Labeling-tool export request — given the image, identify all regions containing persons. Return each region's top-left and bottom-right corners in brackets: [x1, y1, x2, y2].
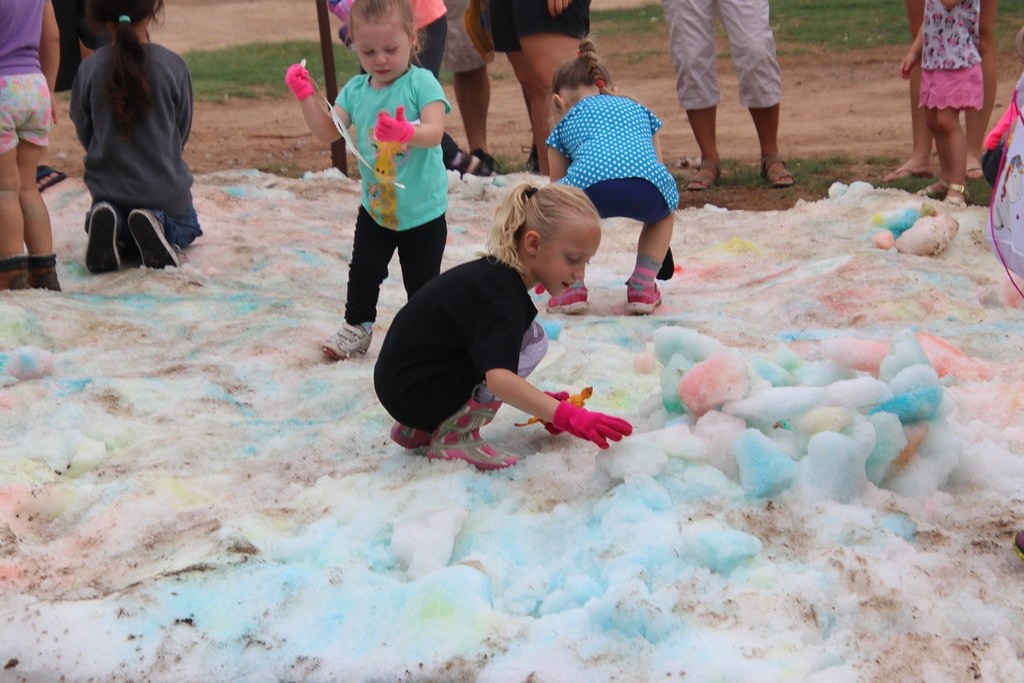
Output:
[410, 0, 593, 177]
[67, 0, 205, 273]
[0, 0, 62, 292]
[882, 0, 999, 183]
[285, 0, 452, 360]
[373, 184, 632, 472]
[545, 40, 680, 313]
[660, 0, 795, 190]
[903, 0, 984, 208]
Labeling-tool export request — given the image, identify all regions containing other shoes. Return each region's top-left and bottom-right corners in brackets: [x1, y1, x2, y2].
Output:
[127, 209, 179, 269]
[522, 144, 538, 172]
[86, 201, 121, 274]
[473, 149, 501, 176]
[0, 254, 28, 289]
[27, 253, 60, 291]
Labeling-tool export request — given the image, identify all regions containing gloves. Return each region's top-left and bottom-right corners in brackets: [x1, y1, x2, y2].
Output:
[285, 64, 316, 101]
[539, 390, 570, 436]
[554, 399, 633, 449]
[376, 105, 415, 144]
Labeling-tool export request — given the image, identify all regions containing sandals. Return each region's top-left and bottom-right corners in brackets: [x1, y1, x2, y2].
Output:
[917, 179, 949, 198]
[945, 185, 967, 206]
[760, 156, 794, 187]
[689, 160, 721, 190]
[36, 165, 65, 191]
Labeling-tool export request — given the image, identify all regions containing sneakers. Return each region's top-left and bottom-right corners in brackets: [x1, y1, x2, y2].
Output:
[627, 282, 661, 314]
[546, 287, 588, 315]
[319, 321, 372, 359]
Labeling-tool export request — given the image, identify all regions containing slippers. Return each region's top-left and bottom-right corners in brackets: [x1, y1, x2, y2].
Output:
[885, 168, 922, 182]
[966, 162, 984, 179]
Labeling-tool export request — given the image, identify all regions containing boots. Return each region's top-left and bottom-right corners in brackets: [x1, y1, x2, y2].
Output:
[428, 398, 518, 470]
[390, 420, 436, 449]
